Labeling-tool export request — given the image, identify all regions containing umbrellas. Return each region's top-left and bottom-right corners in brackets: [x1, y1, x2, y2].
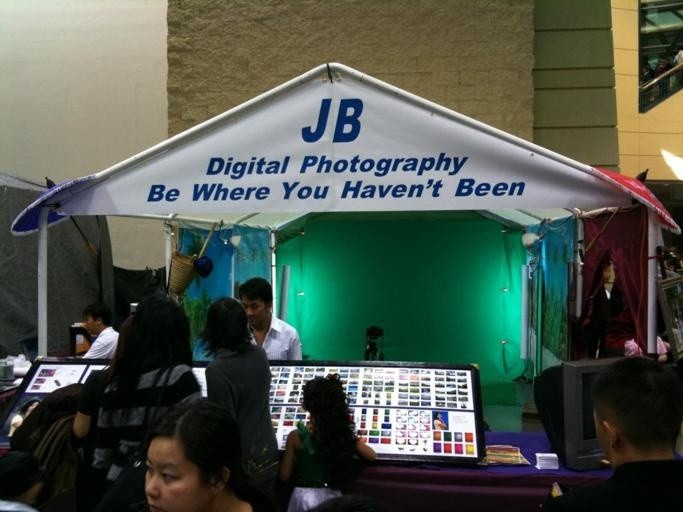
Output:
[580, 155, 680, 262]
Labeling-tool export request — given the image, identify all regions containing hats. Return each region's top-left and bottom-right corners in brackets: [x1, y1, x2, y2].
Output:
[1, 450, 47, 498]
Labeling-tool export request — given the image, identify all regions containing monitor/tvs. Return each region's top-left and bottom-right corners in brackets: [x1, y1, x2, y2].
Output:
[532, 357, 632, 470]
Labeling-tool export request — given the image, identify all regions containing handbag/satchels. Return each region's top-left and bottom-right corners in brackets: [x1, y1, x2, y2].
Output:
[168, 255, 196, 295]
[97, 362, 175, 511]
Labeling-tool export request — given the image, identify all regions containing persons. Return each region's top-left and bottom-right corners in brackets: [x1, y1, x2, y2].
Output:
[431, 411, 448, 431]
[237, 276, 304, 361]
[541, 356, 682, 512]
[73, 293, 203, 512]
[282, 374, 376, 489]
[196, 295, 280, 512]
[134, 394, 281, 512]
[0, 451, 48, 512]
[4, 395, 39, 438]
[77, 301, 120, 361]
